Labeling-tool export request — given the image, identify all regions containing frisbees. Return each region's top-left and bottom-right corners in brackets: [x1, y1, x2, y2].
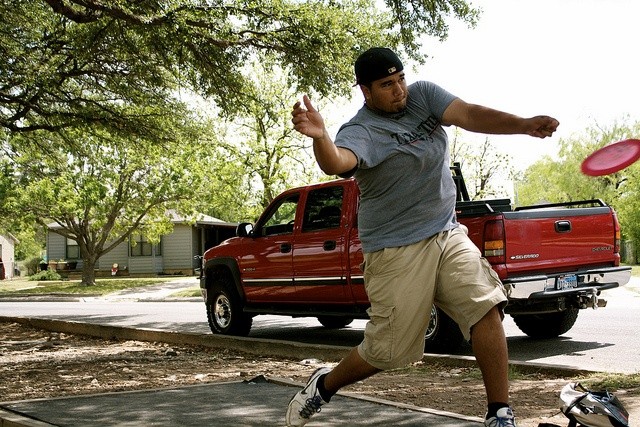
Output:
[580, 138, 640, 176]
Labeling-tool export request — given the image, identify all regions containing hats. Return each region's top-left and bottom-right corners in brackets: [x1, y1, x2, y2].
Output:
[352, 46, 404, 88]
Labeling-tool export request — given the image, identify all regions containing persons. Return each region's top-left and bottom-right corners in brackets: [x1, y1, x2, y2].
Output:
[286, 47, 559, 427]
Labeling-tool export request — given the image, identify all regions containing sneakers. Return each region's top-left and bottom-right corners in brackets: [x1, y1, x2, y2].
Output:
[483, 407, 518, 427]
[285, 364, 332, 426]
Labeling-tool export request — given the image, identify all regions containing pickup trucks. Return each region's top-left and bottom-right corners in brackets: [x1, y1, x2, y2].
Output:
[194, 163, 632, 353]
